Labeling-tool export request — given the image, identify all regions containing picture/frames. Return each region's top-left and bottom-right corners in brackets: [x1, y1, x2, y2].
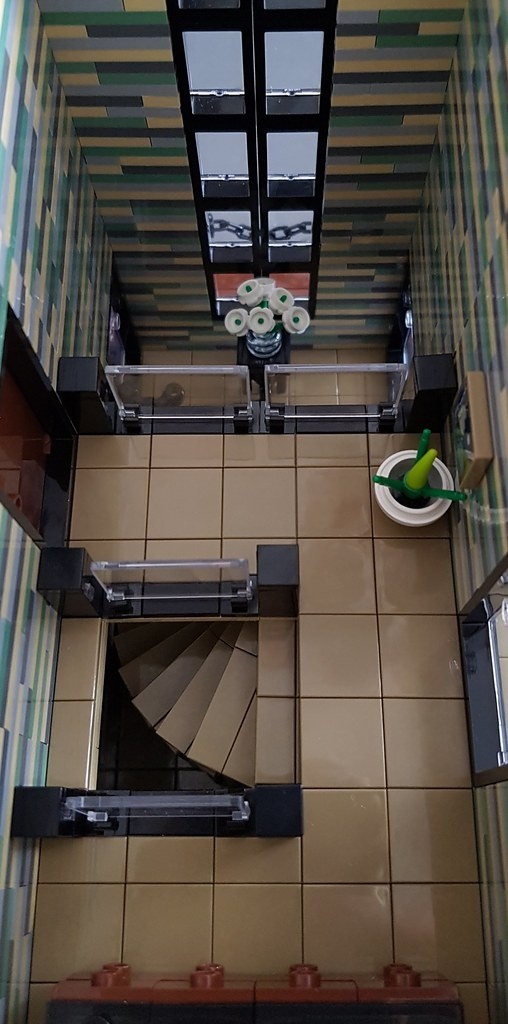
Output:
[451, 369, 494, 490]
